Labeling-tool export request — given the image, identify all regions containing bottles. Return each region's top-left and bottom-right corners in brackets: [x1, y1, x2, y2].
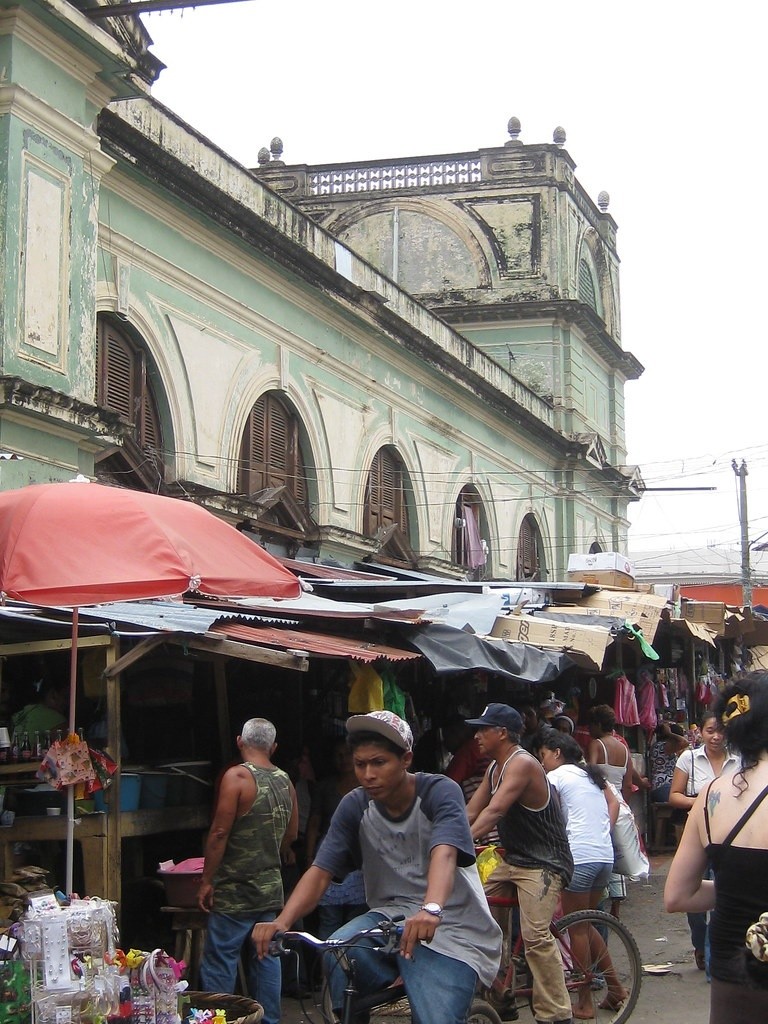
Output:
[33, 731, 41, 762]
[57, 730, 62, 742]
[11, 733, 21, 763]
[42, 730, 50, 761]
[21, 732, 31, 763]
[78, 727, 85, 742]
[0, 747, 11, 764]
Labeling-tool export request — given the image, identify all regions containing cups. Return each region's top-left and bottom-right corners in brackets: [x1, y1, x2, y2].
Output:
[0, 727, 10, 747]
[47, 808, 61, 816]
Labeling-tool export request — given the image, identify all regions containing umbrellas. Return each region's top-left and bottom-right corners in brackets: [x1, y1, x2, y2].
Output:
[3, 473, 301, 894]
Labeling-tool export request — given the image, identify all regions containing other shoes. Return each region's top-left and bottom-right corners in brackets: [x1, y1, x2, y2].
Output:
[495, 1006, 519, 1022]
[695, 947, 705, 970]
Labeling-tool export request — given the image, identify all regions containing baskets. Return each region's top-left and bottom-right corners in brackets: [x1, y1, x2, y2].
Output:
[101, 991, 264, 1024]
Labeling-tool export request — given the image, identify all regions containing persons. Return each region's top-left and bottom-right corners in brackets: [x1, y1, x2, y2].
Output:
[252, 702, 649, 1023]
[196, 718, 300, 1023]
[647, 672, 768, 1023]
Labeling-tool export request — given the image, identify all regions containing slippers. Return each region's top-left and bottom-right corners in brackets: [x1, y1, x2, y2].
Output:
[596, 1000, 626, 1009]
[571, 1004, 595, 1019]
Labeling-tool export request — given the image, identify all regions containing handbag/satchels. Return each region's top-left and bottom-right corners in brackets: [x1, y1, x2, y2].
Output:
[475, 844, 506, 886]
[603, 778, 650, 882]
[694, 677, 713, 706]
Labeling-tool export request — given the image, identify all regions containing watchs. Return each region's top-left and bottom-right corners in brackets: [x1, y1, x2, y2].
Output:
[421, 901, 446, 919]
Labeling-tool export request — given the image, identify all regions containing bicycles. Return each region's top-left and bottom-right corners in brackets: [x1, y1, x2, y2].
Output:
[474, 837, 644, 1024]
[269, 911, 502, 1024]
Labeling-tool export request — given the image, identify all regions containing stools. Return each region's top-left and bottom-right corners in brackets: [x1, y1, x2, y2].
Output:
[652, 802, 677, 855]
[160, 906, 250, 998]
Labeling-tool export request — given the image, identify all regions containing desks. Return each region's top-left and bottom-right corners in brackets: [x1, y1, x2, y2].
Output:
[0, 803, 212, 928]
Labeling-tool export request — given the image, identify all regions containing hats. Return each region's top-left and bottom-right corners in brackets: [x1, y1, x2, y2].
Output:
[346, 709, 414, 751]
[465, 703, 524, 733]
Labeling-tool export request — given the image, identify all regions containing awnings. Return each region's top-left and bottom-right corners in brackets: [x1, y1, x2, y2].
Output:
[2, 585, 768, 673]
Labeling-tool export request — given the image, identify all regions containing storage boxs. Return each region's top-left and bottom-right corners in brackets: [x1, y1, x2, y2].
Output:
[568, 570, 634, 589]
[568, 551, 636, 579]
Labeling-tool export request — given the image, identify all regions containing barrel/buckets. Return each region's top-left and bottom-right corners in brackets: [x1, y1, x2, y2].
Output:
[94, 772, 141, 812]
